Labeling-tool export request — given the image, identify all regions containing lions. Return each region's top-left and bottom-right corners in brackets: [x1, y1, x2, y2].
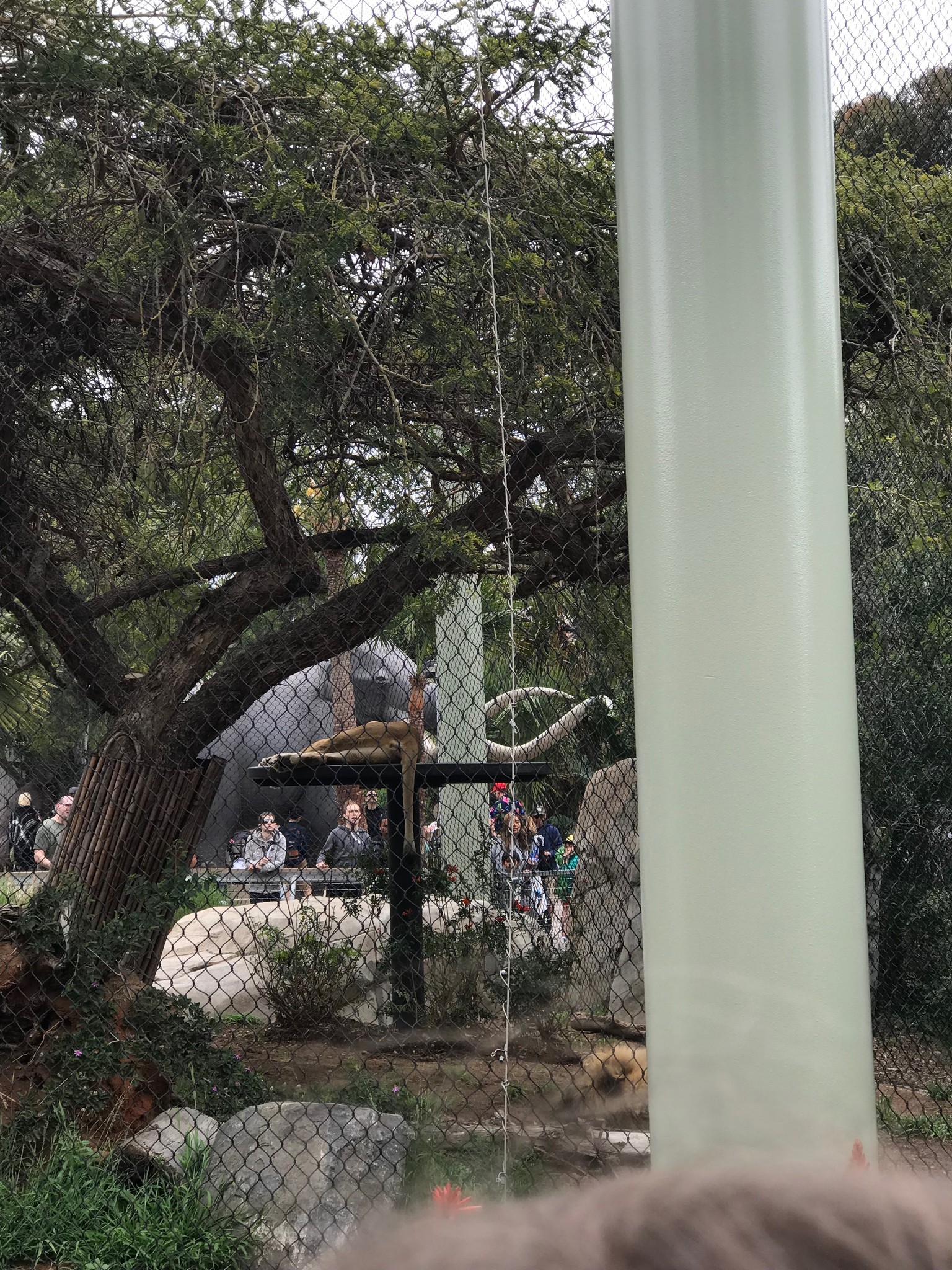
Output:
[258, 721, 437, 866]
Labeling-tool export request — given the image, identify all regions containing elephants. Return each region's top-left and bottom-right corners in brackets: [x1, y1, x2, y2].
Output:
[183, 635, 614, 869]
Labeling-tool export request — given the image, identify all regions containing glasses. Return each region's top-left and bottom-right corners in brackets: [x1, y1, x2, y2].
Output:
[534, 814, 545, 818]
[264, 819, 275, 823]
[367, 794, 378, 797]
[59, 803, 71, 808]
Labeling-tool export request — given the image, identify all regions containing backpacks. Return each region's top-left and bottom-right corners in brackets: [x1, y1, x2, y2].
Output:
[285, 830, 303, 860]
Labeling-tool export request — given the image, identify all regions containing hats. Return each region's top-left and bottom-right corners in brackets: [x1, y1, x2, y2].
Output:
[534, 805, 546, 815]
[563, 835, 573, 844]
[18, 792, 32, 806]
[490, 783, 506, 793]
[69, 786, 78, 797]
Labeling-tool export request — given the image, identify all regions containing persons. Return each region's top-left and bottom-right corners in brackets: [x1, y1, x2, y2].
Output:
[281, 806, 313, 900]
[189, 854, 202, 869]
[244, 811, 286, 904]
[316, 800, 372, 899]
[33, 787, 78, 871]
[8, 792, 43, 872]
[489, 783, 579, 935]
[364, 789, 442, 868]
